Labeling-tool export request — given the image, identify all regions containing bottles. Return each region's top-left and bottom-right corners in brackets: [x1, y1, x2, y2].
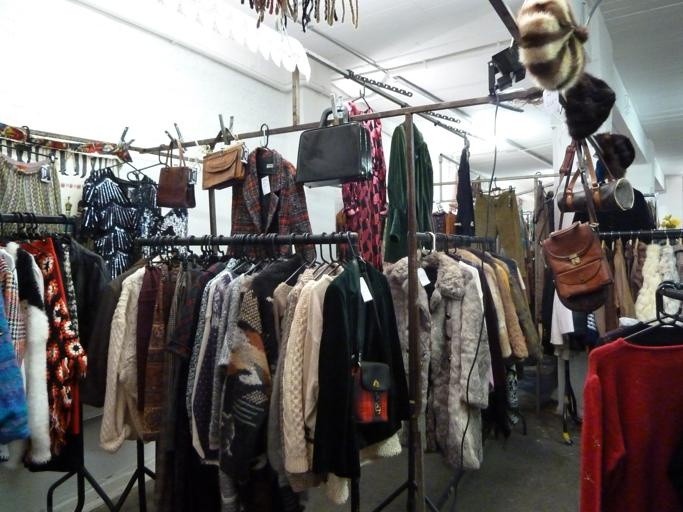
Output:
[594, 135, 635, 178]
[516, 0, 586, 93]
[569, 73, 614, 138]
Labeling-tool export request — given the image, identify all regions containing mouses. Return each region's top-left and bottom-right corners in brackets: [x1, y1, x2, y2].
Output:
[104, 142, 171, 185]
[617, 278, 683, 340]
[131, 234, 366, 288]
[596, 226, 683, 253]
[420, 234, 517, 273]
[252, 123, 274, 164]
[0, 211, 75, 259]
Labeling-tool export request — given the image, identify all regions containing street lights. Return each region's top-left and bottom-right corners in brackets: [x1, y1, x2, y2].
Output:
[294, 107, 373, 188]
[541, 223, 612, 314]
[350, 272, 392, 426]
[157, 139, 196, 206]
[558, 137, 635, 212]
[203, 129, 247, 189]
